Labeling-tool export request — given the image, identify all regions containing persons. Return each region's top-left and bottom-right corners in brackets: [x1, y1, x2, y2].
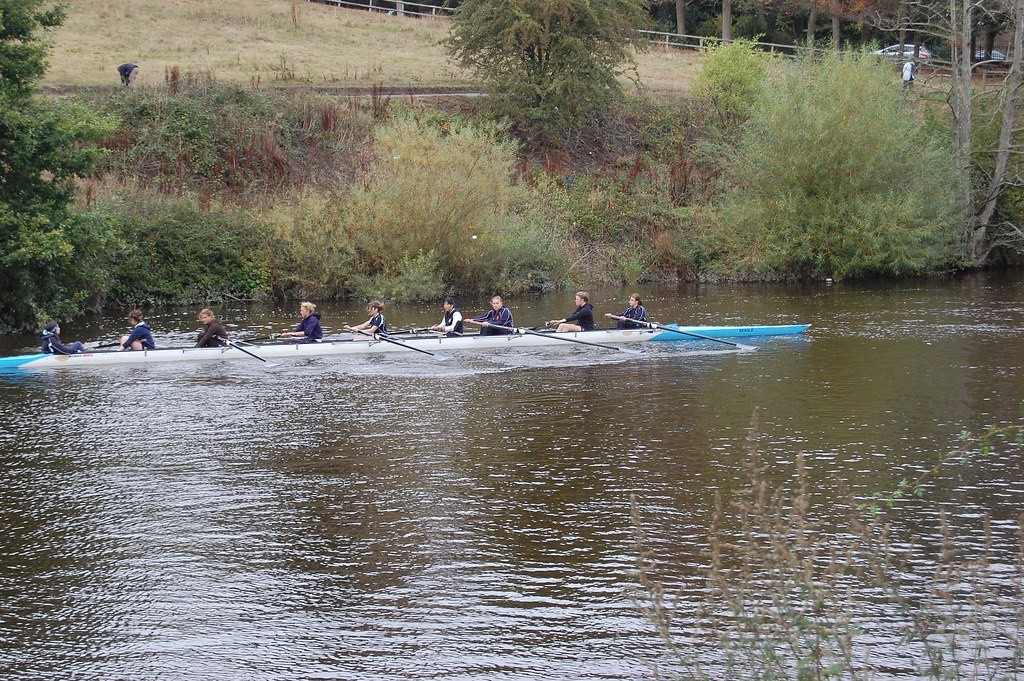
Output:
[350, 300, 388, 342]
[605, 292, 647, 329]
[464, 292, 514, 336]
[901, 56, 915, 92]
[281, 300, 323, 344]
[116, 307, 155, 351]
[41, 321, 87, 353]
[117, 63, 139, 89]
[430, 296, 464, 338]
[194, 307, 229, 348]
[549, 290, 594, 332]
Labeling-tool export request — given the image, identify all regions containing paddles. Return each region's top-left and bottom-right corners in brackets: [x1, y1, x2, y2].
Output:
[234, 334, 281, 343]
[606, 312, 761, 351]
[464, 318, 642, 353]
[388, 325, 439, 335]
[91, 341, 120, 350]
[214, 334, 276, 364]
[524, 320, 555, 329]
[345, 324, 449, 359]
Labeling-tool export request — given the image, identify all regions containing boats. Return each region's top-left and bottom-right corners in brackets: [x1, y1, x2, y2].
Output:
[1, 324, 813, 377]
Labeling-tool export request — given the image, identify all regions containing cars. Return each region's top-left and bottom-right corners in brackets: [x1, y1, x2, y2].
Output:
[870, 44, 932, 65]
[958, 50, 1008, 65]
